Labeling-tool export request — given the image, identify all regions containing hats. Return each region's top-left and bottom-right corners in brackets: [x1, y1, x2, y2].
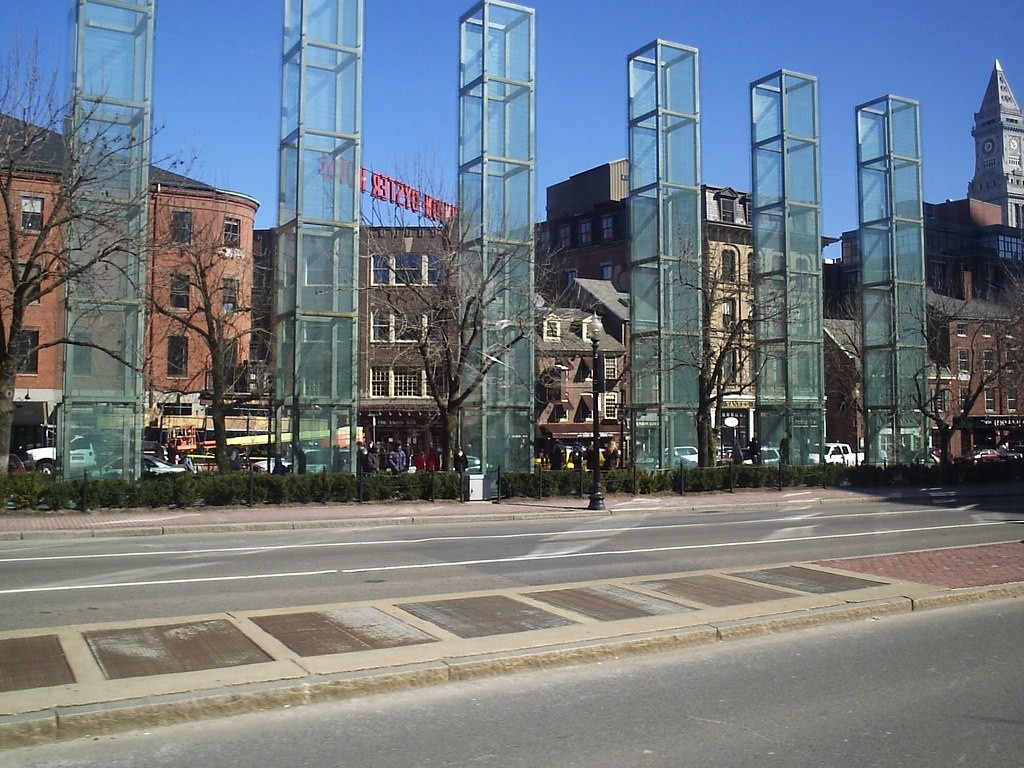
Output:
[393, 440, 401, 449]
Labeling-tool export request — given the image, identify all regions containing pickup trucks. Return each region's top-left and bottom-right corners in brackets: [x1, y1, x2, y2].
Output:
[806, 442, 888, 469]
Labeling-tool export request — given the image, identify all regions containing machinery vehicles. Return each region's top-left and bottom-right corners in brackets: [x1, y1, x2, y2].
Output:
[25, 404, 329, 484]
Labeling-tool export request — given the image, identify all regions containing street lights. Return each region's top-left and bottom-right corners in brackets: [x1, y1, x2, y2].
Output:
[586, 310, 606, 511]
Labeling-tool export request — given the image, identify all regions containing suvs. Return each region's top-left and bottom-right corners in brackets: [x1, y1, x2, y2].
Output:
[252, 449, 351, 477]
[716, 448, 781, 466]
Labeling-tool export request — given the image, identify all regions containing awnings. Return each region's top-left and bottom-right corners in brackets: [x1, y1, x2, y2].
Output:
[11, 401, 49, 425]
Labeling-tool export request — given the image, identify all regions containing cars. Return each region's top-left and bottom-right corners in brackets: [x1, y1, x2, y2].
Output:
[664, 446, 699, 468]
[461, 454, 495, 475]
[8, 454, 26, 474]
[908, 447, 1024, 465]
[92, 456, 188, 477]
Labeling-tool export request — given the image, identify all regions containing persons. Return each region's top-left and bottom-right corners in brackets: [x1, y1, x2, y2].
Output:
[748, 437, 758, 464]
[585, 440, 595, 469]
[548, 441, 567, 470]
[403, 443, 414, 471]
[568, 442, 583, 468]
[273, 460, 286, 473]
[332, 445, 346, 472]
[414, 451, 434, 472]
[453, 446, 468, 473]
[387, 440, 406, 473]
[602, 440, 621, 469]
[800, 437, 810, 465]
[464, 444, 472, 456]
[779, 432, 792, 464]
[356, 441, 387, 472]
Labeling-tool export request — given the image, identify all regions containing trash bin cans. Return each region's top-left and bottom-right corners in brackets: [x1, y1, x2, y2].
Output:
[464, 474, 491, 501]
